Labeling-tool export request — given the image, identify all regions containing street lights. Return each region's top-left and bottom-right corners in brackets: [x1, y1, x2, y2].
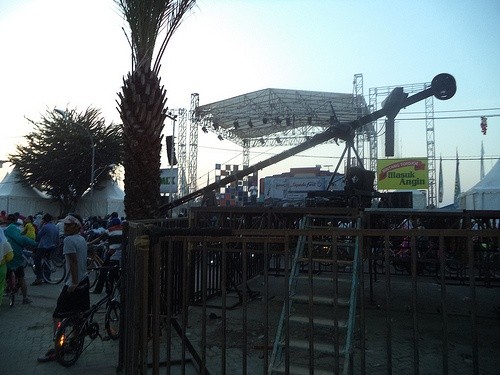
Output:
[54, 108, 96, 217]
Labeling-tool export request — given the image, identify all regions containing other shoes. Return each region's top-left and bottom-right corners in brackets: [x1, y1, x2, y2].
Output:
[22, 296, 32, 304]
[29, 280, 44, 286]
[38, 349, 57, 362]
[90, 289, 101, 294]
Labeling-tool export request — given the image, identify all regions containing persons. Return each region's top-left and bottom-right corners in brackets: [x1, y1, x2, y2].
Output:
[0, 211, 65, 304]
[82, 212, 126, 294]
[248, 183, 258, 204]
[38, 214, 90, 361]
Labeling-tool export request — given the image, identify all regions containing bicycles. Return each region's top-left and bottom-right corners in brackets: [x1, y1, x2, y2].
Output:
[2, 231, 121, 368]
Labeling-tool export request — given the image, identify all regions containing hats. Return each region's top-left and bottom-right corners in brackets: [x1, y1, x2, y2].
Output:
[57, 215, 82, 227]
[109, 218, 120, 225]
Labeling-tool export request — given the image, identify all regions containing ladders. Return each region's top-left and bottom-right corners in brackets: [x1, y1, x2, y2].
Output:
[268, 215, 363, 375]
